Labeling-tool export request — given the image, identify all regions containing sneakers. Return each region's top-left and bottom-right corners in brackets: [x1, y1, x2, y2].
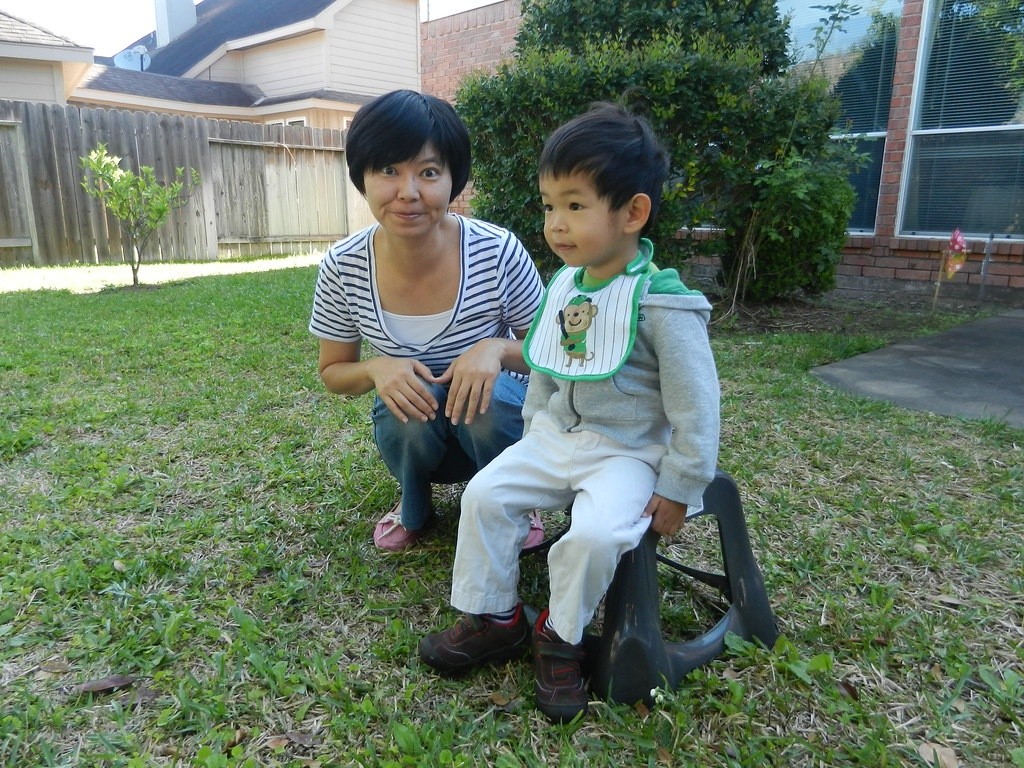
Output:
[533, 609, 587, 725]
[420, 603, 529, 674]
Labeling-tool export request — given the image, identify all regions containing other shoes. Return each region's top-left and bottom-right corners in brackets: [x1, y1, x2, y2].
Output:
[374, 498, 422, 555]
[524, 511, 545, 550]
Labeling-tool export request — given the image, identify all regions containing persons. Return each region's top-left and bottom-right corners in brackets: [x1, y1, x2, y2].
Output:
[416, 100, 720, 720]
[307, 91, 549, 552]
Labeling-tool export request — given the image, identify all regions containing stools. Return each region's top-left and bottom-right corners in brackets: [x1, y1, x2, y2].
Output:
[518, 462, 778, 711]
